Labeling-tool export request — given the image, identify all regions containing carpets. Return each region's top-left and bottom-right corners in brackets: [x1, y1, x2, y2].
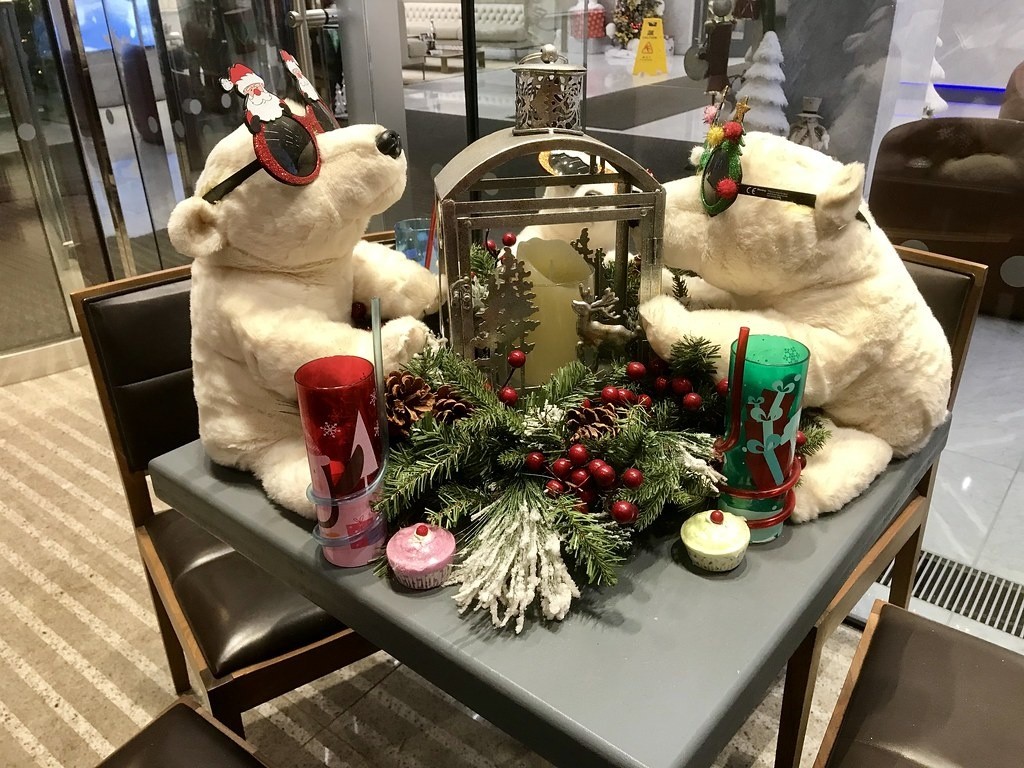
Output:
[505, 62, 763, 131]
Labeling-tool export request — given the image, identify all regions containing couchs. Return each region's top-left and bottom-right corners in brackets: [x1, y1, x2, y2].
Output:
[868, 61, 1024, 320]
[398, 0, 539, 80]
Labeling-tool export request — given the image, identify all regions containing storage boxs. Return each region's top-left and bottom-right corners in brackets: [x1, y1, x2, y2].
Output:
[570, 10, 605, 39]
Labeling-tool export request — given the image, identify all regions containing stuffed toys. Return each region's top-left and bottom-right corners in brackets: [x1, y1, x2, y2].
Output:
[503, 145, 644, 394]
[639, 132, 952, 524]
[168, 98, 448, 525]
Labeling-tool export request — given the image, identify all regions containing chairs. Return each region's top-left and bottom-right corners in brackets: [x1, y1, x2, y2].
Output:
[69, 228, 989, 768]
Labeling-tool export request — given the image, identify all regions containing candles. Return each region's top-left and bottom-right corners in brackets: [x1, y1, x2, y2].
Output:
[508, 237, 595, 386]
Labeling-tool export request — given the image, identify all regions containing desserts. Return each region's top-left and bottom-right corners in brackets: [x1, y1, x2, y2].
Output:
[680, 510, 750, 572]
[386, 522, 456, 589]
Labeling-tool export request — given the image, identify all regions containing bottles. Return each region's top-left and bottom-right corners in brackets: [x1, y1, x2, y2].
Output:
[429, 20, 436, 48]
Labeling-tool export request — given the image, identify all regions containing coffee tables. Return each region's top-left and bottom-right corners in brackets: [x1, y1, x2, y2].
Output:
[424, 49, 485, 74]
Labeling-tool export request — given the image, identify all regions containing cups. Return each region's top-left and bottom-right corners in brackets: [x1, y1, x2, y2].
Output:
[294, 355, 389, 566]
[720, 334, 812, 546]
[393, 217, 437, 271]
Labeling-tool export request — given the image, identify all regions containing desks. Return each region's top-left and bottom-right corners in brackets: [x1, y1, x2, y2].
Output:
[148, 410, 953, 768]
